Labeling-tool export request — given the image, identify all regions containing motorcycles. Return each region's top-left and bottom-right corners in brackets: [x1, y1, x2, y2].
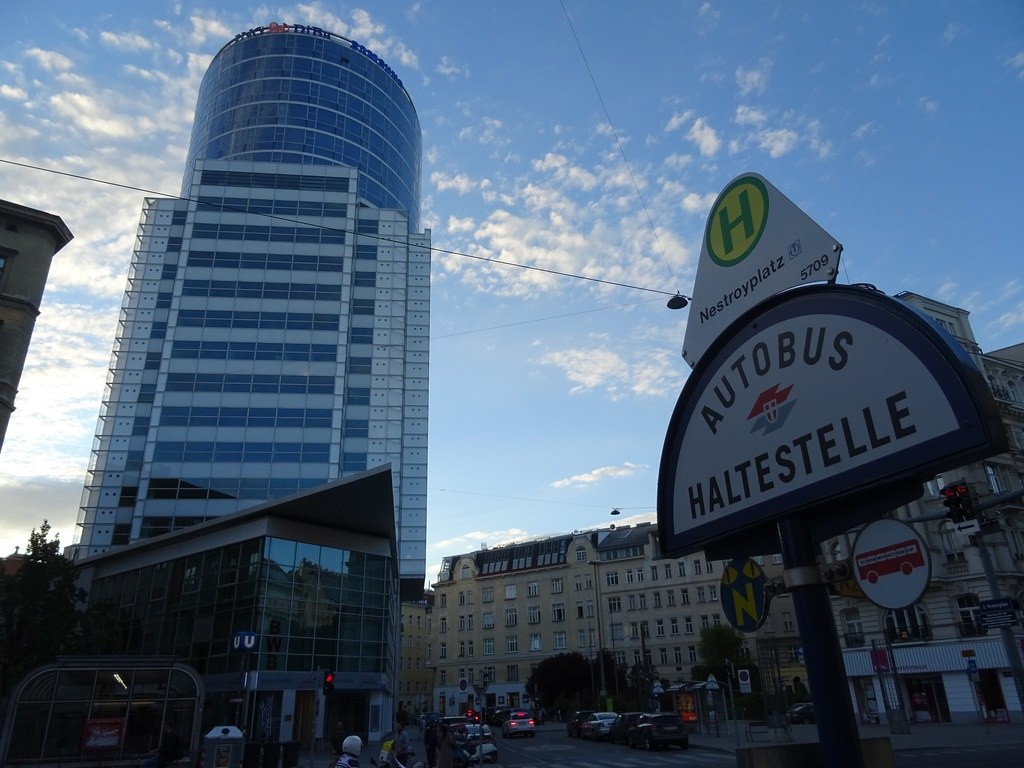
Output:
[348, 740, 388, 768]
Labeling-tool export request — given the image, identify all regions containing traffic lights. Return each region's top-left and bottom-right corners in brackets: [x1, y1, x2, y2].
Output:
[323, 670, 336, 695]
[939, 484, 966, 524]
[466, 708, 473, 719]
[950, 476, 982, 522]
[474, 714, 479, 723]
[485, 707, 496, 720]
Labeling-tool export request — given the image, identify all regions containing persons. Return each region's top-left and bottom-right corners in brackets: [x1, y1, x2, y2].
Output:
[258, 735, 284, 768]
[142, 718, 181, 768]
[329, 718, 488, 768]
[538, 708, 547, 725]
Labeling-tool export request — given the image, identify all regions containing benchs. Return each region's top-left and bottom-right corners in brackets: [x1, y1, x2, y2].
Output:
[707, 720, 723, 734]
[745, 719, 792, 743]
[684, 721, 701, 734]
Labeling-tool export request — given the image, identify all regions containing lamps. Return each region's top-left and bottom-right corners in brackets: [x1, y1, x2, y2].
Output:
[666, 295, 692, 310]
[611, 509, 620, 515]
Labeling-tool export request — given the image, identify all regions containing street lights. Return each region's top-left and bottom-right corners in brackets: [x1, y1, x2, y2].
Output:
[586, 560, 606, 698]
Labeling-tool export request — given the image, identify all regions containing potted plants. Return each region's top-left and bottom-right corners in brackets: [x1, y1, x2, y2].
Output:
[913, 693, 922, 703]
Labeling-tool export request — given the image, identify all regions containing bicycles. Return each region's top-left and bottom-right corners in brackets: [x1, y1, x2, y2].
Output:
[379, 734, 427, 768]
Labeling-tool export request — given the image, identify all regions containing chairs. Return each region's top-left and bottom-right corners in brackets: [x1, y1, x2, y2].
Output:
[899, 631, 911, 640]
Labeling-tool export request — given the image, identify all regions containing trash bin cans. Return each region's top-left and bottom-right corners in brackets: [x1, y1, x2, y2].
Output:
[202, 726, 248, 768]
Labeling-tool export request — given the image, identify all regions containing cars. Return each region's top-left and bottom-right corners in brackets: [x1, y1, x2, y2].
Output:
[580, 712, 621, 741]
[607, 710, 648, 745]
[787, 702, 817, 725]
[566, 710, 601, 738]
[417, 712, 498, 765]
[624, 712, 690, 751]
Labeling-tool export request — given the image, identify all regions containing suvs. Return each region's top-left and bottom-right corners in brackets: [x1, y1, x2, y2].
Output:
[502, 711, 536, 739]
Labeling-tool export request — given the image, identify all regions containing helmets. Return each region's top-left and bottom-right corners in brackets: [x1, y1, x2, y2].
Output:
[343, 735, 363, 757]
